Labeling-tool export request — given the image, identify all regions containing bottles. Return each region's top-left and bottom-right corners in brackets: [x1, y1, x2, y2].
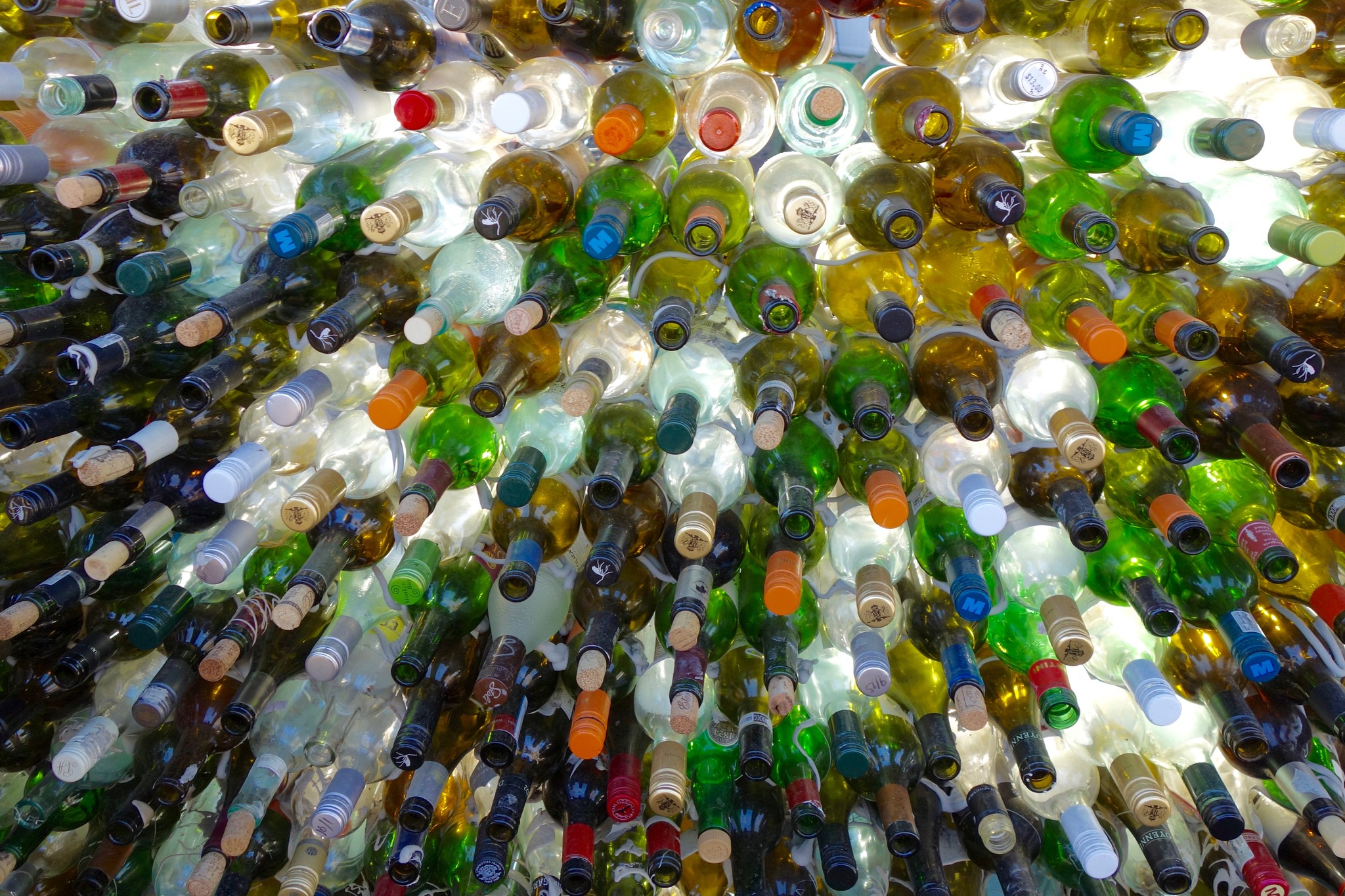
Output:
[1, 1, 1344, 896]
